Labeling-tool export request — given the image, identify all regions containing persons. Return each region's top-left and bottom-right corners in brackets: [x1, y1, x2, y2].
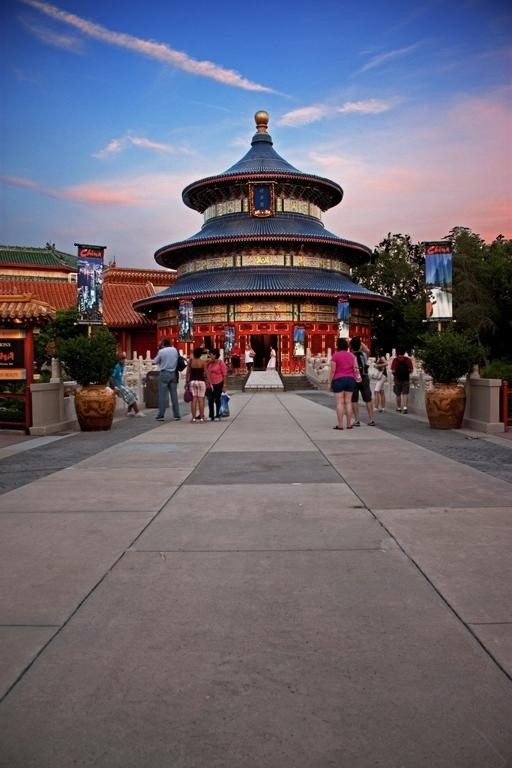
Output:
[265, 344, 277, 370]
[204, 349, 227, 420]
[390, 348, 414, 414]
[153, 338, 181, 420]
[185, 347, 213, 422]
[328, 339, 357, 429]
[371, 346, 389, 412]
[231, 341, 242, 376]
[109, 351, 145, 418]
[346, 337, 376, 426]
[244, 343, 256, 372]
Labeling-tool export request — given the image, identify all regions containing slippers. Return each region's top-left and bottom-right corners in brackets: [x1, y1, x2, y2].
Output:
[334, 424, 344, 431]
[346, 425, 354, 429]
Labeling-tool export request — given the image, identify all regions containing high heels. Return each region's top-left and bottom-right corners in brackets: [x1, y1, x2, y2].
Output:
[373, 408, 379, 413]
[381, 409, 385, 413]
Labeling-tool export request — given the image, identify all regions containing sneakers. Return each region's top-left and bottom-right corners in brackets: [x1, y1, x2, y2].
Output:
[172, 416, 181, 421]
[191, 416, 222, 422]
[403, 407, 408, 413]
[395, 408, 402, 412]
[155, 416, 166, 421]
[135, 410, 147, 417]
[368, 419, 376, 427]
[126, 411, 135, 417]
[352, 420, 361, 427]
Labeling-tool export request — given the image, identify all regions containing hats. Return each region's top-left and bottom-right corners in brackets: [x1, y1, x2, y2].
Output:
[117, 351, 127, 360]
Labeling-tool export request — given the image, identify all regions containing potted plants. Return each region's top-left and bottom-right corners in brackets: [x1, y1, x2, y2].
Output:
[413, 328, 483, 430]
[56, 322, 117, 433]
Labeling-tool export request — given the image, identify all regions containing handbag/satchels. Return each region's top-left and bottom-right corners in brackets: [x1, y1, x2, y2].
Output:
[183, 386, 193, 403]
[354, 361, 363, 383]
[219, 392, 230, 418]
[249, 353, 256, 357]
[178, 353, 188, 371]
[372, 369, 383, 379]
